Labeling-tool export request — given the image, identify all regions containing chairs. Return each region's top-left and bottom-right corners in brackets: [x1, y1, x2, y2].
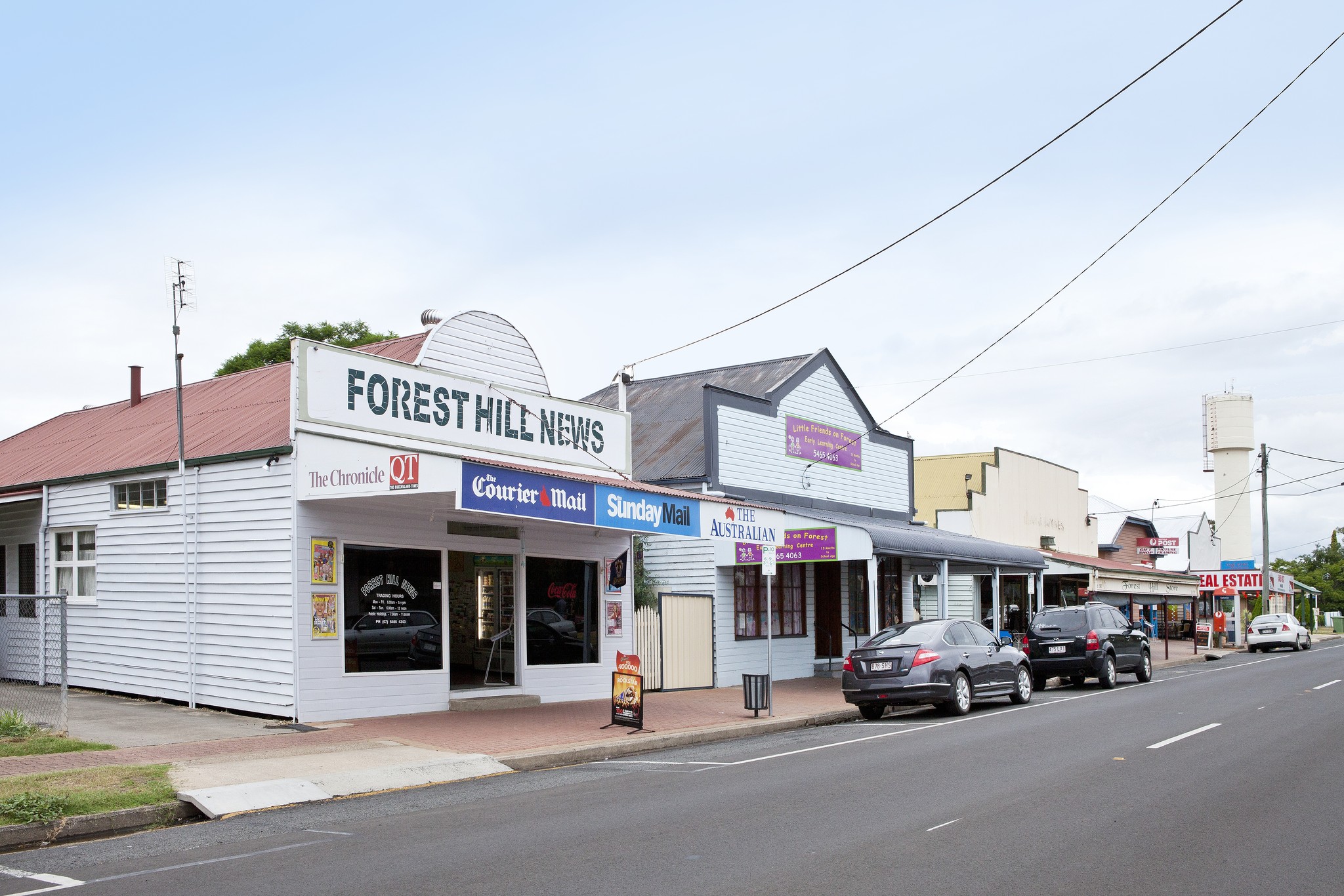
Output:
[1178, 623, 1191, 641]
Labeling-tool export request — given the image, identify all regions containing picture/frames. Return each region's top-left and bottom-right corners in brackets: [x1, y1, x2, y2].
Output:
[309, 534, 338, 586]
[611, 671, 644, 730]
[309, 591, 339, 641]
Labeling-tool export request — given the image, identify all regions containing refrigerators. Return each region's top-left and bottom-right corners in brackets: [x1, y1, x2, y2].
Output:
[473, 555, 514, 673]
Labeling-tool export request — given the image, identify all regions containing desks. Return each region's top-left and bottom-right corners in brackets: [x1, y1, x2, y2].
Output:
[1157, 623, 1182, 638]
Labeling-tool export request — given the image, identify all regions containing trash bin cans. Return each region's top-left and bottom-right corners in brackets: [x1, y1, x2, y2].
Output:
[1330, 616, 1344, 633]
[742, 673, 770, 710]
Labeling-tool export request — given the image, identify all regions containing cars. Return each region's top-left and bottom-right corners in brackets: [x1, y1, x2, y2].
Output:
[1246, 613, 1311, 653]
[525, 608, 579, 640]
[408, 615, 595, 672]
[345, 611, 440, 658]
[981, 604, 1037, 624]
[839, 617, 1033, 721]
[576, 611, 595, 631]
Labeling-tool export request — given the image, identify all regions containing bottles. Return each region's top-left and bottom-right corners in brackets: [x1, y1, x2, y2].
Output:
[482, 595, 493, 638]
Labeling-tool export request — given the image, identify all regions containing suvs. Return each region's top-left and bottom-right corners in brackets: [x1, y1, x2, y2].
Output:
[1021, 602, 1152, 692]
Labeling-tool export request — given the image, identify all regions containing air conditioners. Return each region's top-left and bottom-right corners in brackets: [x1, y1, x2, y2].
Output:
[917, 575, 938, 586]
